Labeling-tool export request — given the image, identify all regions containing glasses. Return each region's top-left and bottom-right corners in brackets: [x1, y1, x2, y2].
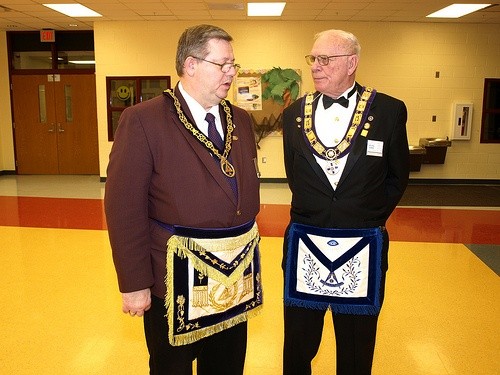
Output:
[185, 55, 240, 74]
[305, 54, 352, 65]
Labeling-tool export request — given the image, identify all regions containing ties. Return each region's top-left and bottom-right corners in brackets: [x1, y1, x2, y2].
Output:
[205, 114, 238, 200]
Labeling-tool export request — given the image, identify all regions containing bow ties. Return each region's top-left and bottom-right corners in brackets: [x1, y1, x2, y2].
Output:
[323, 85, 357, 109]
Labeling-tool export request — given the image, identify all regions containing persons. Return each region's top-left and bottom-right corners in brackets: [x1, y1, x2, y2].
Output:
[280, 29, 411, 375]
[104, 24, 264, 375]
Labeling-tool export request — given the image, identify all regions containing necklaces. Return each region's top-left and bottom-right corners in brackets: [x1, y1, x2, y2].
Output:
[301, 86, 377, 175]
[163, 88, 236, 178]
